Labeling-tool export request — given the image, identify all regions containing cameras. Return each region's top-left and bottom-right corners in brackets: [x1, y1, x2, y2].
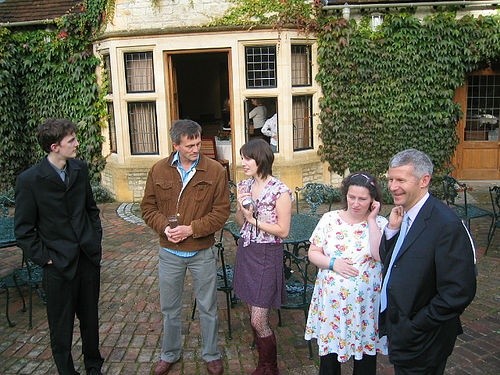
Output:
[242, 198, 257, 213]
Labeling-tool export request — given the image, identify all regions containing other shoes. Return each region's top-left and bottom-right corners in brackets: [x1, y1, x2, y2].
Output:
[86, 367, 102, 375]
[206, 359, 223, 375]
[152, 360, 171, 375]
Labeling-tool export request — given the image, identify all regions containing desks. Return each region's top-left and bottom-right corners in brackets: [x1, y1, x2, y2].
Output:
[215, 215, 327, 359]
[0, 216, 44, 329]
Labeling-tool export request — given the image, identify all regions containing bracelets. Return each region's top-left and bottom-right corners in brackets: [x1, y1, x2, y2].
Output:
[329, 257, 336, 271]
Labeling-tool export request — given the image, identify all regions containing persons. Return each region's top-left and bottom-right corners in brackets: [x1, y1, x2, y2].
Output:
[140, 119, 231, 375]
[305, 171, 388, 375]
[378, 149, 477, 375]
[261, 113, 278, 152]
[232, 138, 294, 375]
[15, 118, 105, 375]
[248, 99, 268, 137]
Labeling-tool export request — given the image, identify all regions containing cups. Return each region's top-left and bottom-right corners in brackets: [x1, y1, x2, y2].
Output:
[169, 219, 178, 228]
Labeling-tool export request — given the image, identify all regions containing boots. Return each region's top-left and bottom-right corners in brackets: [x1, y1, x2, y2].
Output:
[251, 331, 279, 375]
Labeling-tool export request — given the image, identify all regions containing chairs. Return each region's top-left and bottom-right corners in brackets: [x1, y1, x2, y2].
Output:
[0, 252, 49, 330]
[294, 182, 338, 273]
[442, 176, 494, 241]
[484, 185, 500, 255]
[192, 243, 235, 339]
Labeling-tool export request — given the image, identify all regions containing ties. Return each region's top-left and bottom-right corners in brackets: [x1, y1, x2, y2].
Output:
[381, 214, 409, 313]
